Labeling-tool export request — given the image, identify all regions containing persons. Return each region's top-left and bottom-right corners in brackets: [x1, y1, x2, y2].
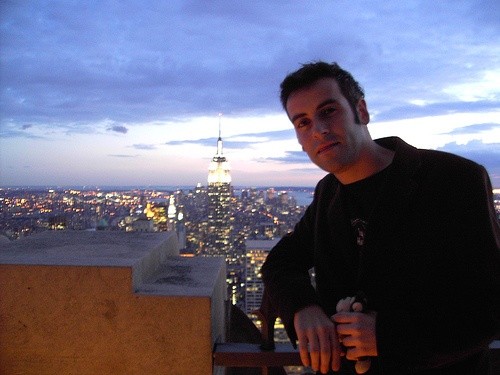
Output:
[260, 59, 500, 375]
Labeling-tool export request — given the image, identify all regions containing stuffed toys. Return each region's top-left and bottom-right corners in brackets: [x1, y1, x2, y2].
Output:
[336, 296, 373, 374]
